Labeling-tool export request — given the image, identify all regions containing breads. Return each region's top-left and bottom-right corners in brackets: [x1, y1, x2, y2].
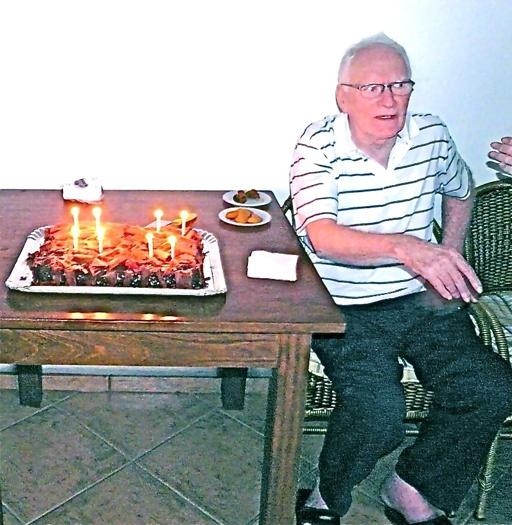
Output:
[227, 208, 261, 225]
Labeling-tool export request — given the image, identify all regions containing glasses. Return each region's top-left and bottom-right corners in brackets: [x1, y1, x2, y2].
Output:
[339, 78, 416, 99]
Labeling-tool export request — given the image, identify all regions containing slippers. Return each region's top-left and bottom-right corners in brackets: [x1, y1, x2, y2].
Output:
[296, 487, 341, 524]
[385, 506, 457, 524]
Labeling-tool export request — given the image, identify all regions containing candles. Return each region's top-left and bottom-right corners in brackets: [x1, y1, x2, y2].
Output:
[70, 205, 188, 259]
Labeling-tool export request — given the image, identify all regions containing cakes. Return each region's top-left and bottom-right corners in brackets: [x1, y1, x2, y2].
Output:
[61, 177, 102, 201]
[32, 223, 207, 291]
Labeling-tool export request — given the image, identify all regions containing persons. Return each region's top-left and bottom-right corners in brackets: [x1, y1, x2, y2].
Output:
[287, 29, 511, 525]
[488, 133, 511, 174]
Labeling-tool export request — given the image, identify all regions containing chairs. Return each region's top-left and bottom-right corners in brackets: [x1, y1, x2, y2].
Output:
[281, 193, 492, 525]
[458, 181, 511, 520]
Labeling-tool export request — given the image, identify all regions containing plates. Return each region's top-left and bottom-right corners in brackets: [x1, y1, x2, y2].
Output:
[217, 206, 273, 228]
[222, 189, 274, 208]
